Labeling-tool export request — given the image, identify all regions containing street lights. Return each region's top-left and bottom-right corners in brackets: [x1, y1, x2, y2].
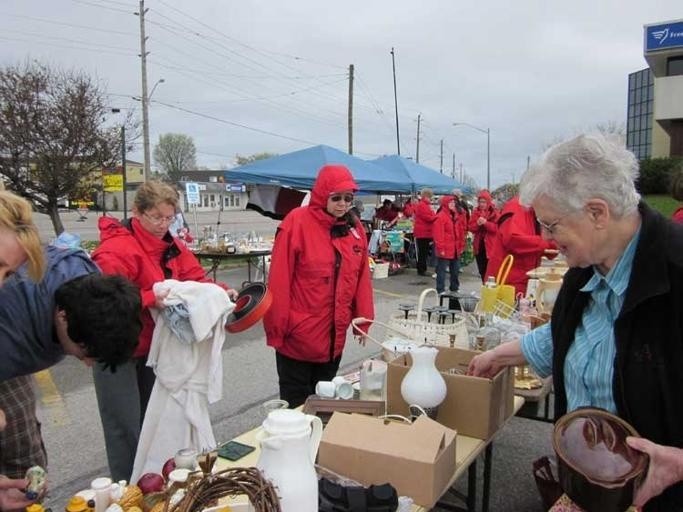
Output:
[140, 75, 166, 184]
[453, 122, 491, 193]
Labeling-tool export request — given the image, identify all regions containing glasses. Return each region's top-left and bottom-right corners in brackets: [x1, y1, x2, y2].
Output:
[140, 208, 176, 226]
[329, 193, 352, 203]
[536, 203, 584, 232]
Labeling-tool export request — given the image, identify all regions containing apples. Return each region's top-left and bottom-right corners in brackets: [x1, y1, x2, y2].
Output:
[136, 473, 164, 495]
[162, 458, 176, 481]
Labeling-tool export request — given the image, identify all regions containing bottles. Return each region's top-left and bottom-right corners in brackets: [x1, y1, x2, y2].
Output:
[25, 465, 49, 502]
[537, 267, 564, 315]
[200, 224, 270, 253]
[486, 276, 496, 288]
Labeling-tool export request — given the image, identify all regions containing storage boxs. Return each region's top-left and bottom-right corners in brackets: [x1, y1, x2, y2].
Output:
[368, 261, 390, 279]
[385, 341, 515, 441]
[315, 404, 461, 507]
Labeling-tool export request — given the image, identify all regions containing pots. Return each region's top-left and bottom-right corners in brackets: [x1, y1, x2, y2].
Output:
[551, 403, 648, 510]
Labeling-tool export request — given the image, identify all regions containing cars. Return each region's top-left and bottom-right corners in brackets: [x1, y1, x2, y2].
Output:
[23, 180, 70, 211]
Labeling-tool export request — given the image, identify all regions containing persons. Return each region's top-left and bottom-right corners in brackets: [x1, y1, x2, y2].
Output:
[0, 230, 144, 511]
[258, 161, 376, 414]
[0, 177, 49, 512]
[461, 126, 682, 511]
[375, 189, 563, 309]
[87, 177, 241, 488]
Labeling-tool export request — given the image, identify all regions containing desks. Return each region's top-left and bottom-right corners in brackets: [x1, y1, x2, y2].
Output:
[516, 367, 555, 421]
[180, 248, 272, 291]
[158, 385, 526, 512]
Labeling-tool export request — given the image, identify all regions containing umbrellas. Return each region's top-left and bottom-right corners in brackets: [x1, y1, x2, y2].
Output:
[372, 153, 472, 195]
[222, 144, 412, 194]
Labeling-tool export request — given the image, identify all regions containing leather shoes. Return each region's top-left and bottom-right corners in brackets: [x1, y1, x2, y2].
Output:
[419, 269, 433, 277]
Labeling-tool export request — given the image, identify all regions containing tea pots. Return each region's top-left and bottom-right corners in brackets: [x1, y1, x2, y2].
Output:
[248, 408, 323, 512]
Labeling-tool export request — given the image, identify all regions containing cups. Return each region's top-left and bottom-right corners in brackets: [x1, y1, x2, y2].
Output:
[64, 478, 125, 512]
[313, 381, 337, 400]
[466, 299, 543, 384]
[331, 376, 353, 400]
[360, 360, 387, 401]
